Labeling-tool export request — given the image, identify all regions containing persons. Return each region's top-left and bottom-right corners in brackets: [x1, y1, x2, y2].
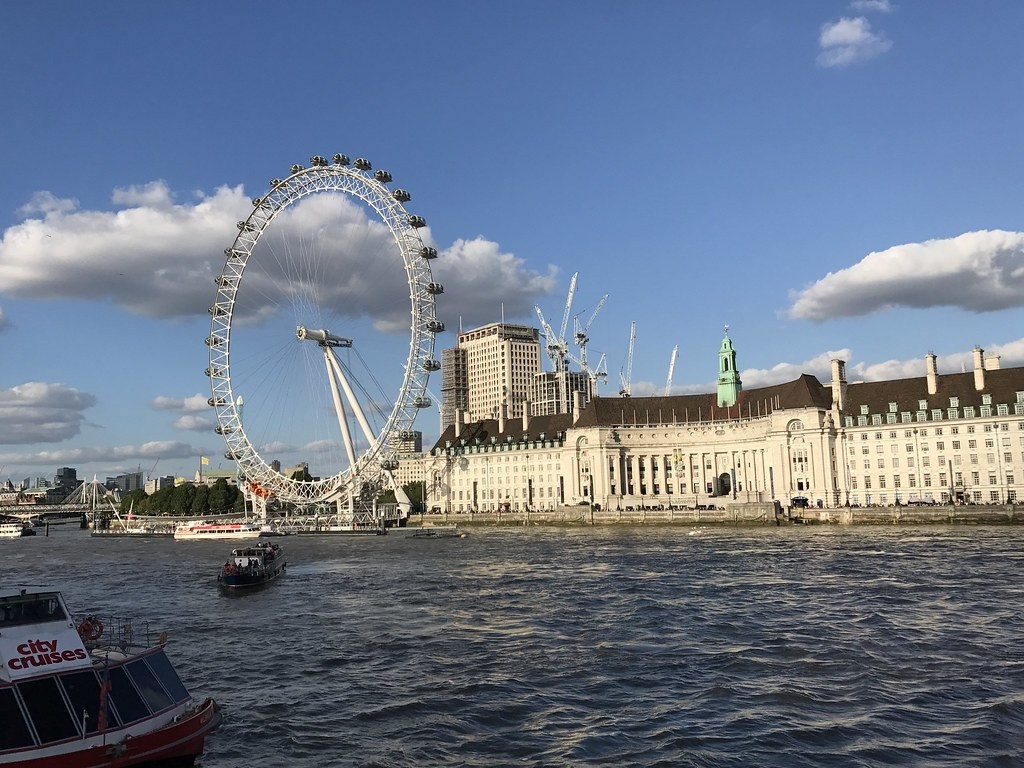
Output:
[226, 558, 258, 574]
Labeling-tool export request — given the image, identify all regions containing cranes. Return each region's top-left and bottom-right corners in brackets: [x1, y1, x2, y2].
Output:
[665, 344, 678, 397]
[534, 271, 610, 397]
[619, 321, 635, 397]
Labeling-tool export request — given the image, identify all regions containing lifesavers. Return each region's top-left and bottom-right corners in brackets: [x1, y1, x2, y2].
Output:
[88, 621, 103, 641]
[79, 621, 93, 643]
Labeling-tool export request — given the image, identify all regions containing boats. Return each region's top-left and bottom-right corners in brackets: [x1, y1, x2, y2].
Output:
[0, 523, 26, 537]
[0, 586, 222, 768]
[174, 523, 262, 540]
[218, 542, 287, 588]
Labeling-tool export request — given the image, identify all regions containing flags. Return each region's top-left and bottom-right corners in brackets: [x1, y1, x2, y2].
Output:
[201, 457, 208, 465]
